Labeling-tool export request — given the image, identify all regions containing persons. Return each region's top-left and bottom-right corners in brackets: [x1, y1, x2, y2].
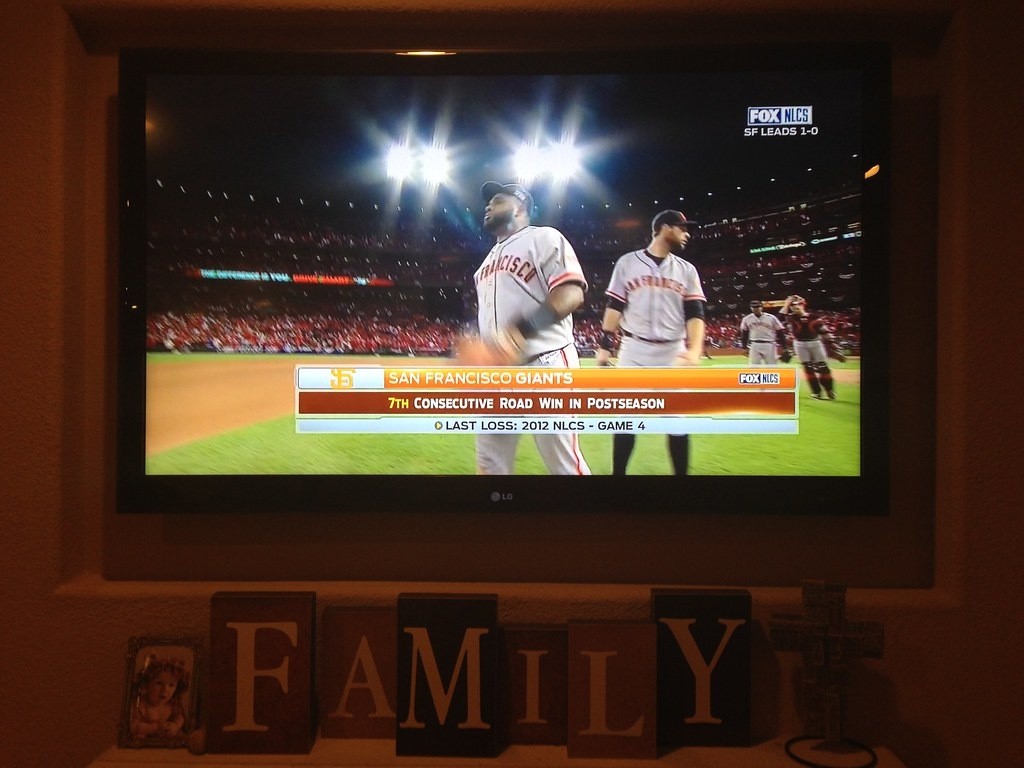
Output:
[818, 309, 861, 349]
[130, 659, 188, 738]
[458, 180, 593, 474]
[777, 295, 842, 399]
[572, 319, 602, 348]
[740, 300, 793, 365]
[598, 209, 707, 475]
[704, 314, 742, 348]
[146, 311, 459, 355]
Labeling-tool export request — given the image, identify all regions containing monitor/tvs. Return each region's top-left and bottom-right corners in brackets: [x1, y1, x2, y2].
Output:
[114, 40, 892, 517]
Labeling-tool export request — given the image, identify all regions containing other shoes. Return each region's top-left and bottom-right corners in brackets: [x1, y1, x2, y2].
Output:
[811, 392, 820, 399]
[827, 389, 837, 400]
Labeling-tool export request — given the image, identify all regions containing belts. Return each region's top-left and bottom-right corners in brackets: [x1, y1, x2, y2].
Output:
[625, 330, 659, 344]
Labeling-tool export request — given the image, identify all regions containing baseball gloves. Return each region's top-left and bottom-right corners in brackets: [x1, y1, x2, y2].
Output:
[777, 351, 793, 364]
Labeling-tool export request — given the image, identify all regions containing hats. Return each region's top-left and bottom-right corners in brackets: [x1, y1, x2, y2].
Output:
[651, 210, 699, 232]
[750, 300, 762, 306]
[479, 180, 533, 217]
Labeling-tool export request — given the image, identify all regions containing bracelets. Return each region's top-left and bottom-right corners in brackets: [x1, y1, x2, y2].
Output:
[599, 329, 615, 351]
